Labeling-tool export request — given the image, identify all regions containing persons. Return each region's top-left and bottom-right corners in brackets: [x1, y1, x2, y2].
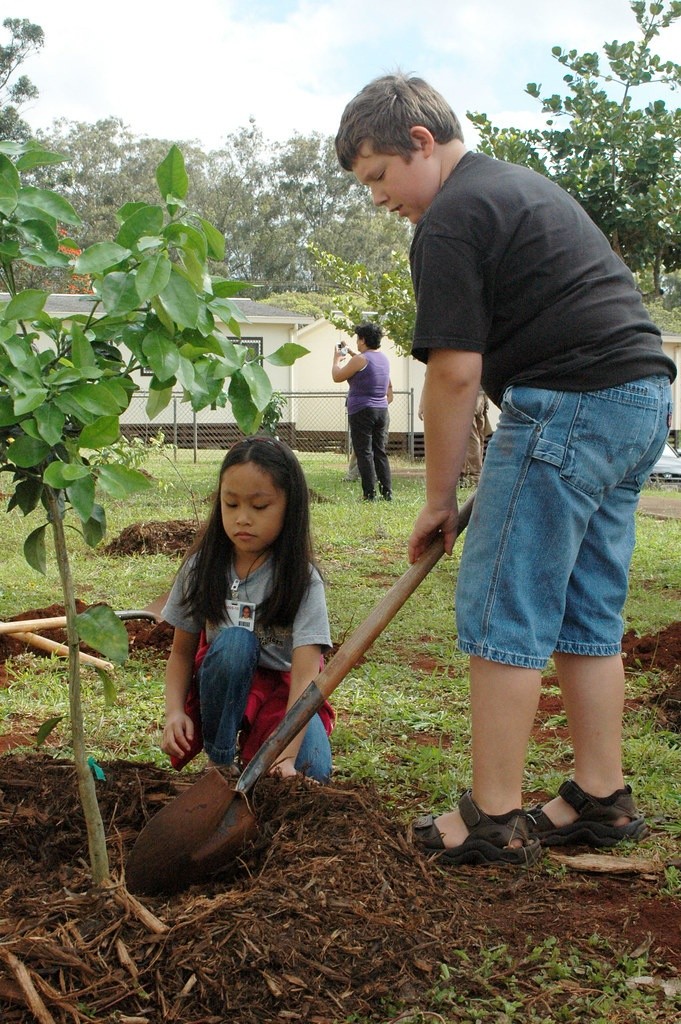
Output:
[157, 437, 335, 785]
[331, 71, 681, 860]
[330, 322, 394, 502]
[417, 369, 493, 487]
[239, 605, 253, 620]
[341, 379, 394, 482]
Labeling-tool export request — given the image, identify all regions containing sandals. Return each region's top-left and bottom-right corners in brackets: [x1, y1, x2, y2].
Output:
[524, 780, 648, 855]
[408, 788, 541, 872]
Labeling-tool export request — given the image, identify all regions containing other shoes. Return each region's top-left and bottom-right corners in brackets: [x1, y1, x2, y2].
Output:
[379, 497, 390, 501]
[356, 496, 371, 502]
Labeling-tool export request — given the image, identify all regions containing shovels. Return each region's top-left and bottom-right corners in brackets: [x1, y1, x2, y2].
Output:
[119, 484, 484, 899]
[0, 585, 182, 672]
